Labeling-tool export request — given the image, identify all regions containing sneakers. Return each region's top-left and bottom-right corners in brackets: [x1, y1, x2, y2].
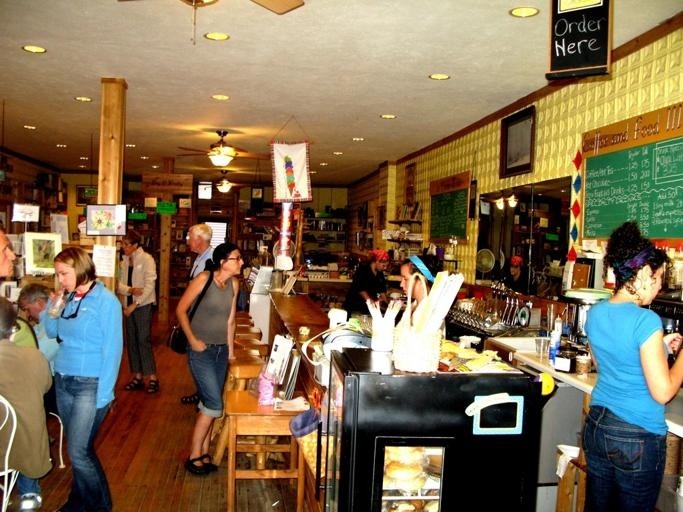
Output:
[19, 493, 44, 512]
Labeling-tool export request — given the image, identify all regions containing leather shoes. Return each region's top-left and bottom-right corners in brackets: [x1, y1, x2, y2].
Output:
[181, 393, 199, 404]
[202, 454, 218, 472]
[184, 455, 208, 475]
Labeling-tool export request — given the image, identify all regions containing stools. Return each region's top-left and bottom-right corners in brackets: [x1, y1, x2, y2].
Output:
[210, 309, 310, 512]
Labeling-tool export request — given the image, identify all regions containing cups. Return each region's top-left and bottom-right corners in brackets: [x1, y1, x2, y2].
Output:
[370, 319, 394, 351]
[354, 215, 374, 250]
[298, 326, 310, 335]
[302, 270, 340, 279]
[392, 327, 441, 372]
[534, 338, 550, 354]
[236, 239, 248, 251]
[306, 219, 346, 233]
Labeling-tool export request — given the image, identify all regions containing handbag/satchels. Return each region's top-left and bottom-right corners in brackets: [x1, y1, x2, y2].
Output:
[167, 326, 188, 354]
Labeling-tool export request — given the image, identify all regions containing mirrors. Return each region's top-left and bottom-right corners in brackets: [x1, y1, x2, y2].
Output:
[474, 176, 571, 302]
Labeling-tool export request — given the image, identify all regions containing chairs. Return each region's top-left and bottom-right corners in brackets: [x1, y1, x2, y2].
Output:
[0, 394, 21, 512]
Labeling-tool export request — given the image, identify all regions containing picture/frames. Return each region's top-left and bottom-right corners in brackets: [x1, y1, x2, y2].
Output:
[75, 185, 97, 206]
[85, 203, 127, 237]
[497, 105, 535, 180]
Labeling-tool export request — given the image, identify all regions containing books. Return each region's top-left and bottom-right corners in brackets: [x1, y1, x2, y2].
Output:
[261, 333, 295, 385]
[280, 346, 302, 401]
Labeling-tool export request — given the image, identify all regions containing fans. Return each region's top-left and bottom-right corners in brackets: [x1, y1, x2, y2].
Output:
[475, 248, 495, 287]
[175, 129, 266, 194]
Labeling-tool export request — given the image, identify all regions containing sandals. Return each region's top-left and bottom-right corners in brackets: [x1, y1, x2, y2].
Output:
[124, 376, 145, 391]
[147, 378, 160, 393]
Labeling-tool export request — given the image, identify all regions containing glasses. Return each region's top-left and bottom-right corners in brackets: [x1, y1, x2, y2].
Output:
[60, 298, 81, 319]
[228, 256, 240, 262]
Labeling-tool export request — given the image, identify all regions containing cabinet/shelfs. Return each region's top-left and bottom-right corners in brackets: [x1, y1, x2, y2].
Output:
[237, 213, 348, 267]
[385, 220, 424, 260]
[0, 172, 67, 231]
[126, 197, 195, 300]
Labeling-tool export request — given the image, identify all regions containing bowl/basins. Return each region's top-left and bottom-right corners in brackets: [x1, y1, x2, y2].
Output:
[443, 254, 455, 260]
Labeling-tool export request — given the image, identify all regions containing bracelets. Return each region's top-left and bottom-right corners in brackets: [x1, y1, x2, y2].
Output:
[135, 301, 139, 306]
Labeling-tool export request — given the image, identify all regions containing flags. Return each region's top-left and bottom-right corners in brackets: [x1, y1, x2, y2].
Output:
[267, 137, 314, 206]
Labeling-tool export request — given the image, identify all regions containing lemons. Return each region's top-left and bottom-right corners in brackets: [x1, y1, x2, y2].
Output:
[535, 372, 555, 396]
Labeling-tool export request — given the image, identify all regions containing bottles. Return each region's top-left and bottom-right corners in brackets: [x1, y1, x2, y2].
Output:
[604, 267, 615, 288]
[360, 314, 369, 329]
[320, 293, 342, 308]
[548, 313, 593, 376]
[448, 296, 530, 336]
[46, 287, 67, 318]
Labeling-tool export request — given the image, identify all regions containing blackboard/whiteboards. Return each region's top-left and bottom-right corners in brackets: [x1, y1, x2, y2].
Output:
[429, 170, 471, 244]
[578, 101, 683, 250]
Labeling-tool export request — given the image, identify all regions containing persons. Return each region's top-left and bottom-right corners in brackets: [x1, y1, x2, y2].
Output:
[582, 222, 682, 510]
[0, 228, 18, 281]
[0, 297, 53, 511]
[500, 255, 538, 297]
[175, 243, 244, 477]
[398, 253, 447, 341]
[16, 283, 57, 416]
[340, 249, 390, 318]
[41, 246, 124, 511]
[178, 223, 216, 411]
[117, 230, 161, 395]
